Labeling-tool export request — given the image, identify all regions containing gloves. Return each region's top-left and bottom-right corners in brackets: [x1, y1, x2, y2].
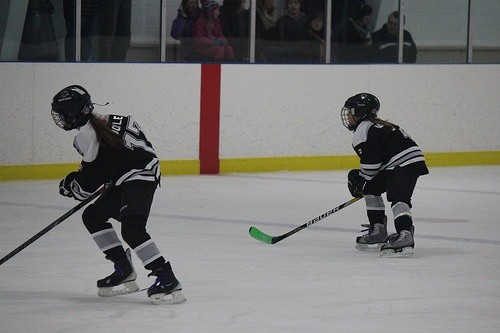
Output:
[347, 168, 371, 200]
[59, 171, 79, 198]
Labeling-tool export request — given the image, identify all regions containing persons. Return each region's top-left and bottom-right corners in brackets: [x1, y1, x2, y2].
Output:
[51, 85, 186, 305]
[171, 0, 336, 63]
[341, 93, 429, 257]
[335, 0, 379, 64]
[371, 11, 418, 64]
[63, 0, 132, 62]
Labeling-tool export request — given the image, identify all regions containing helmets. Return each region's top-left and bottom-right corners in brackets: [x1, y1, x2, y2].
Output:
[345, 93, 381, 132]
[51, 85, 93, 130]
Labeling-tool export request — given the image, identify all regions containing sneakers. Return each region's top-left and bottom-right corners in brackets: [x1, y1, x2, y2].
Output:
[355, 215, 389, 250]
[146, 261, 187, 305]
[377, 225, 415, 259]
[97, 247, 140, 296]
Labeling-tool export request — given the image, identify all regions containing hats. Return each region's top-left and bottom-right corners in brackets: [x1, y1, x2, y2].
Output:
[200, 0, 220, 17]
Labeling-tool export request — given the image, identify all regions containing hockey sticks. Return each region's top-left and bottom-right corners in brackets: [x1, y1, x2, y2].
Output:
[0, 185, 107, 263]
[247, 191, 366, 245]
